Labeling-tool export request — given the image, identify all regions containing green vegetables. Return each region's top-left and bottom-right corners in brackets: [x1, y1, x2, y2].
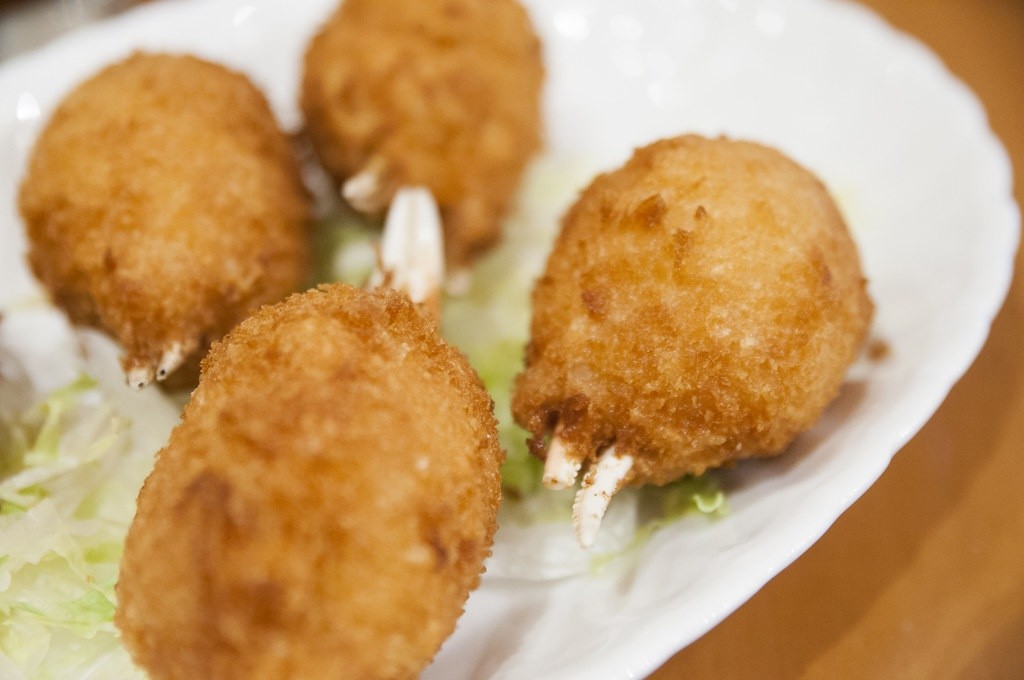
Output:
[0, 211, 728, 679]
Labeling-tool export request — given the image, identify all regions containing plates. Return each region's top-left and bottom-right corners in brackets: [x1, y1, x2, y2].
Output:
[0, 1, 1021, 680]
